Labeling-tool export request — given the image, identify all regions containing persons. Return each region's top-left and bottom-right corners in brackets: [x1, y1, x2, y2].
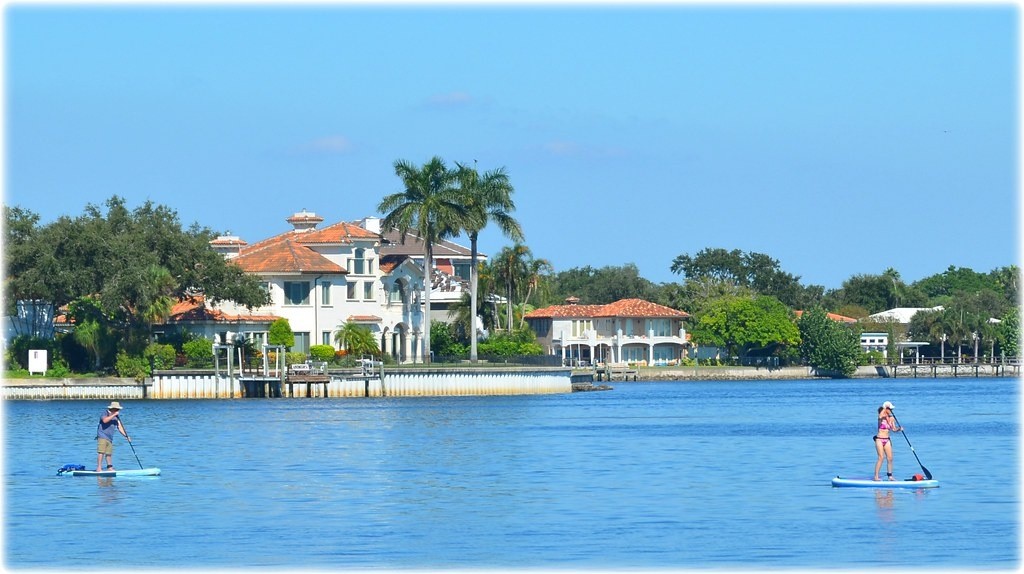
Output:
[97, 401, 132, 473]
[874, 401, 904, 481]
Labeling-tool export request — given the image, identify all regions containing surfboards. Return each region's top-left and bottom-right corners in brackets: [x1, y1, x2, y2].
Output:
[831, 475, 939, 488]
[57, 468, 161, 476]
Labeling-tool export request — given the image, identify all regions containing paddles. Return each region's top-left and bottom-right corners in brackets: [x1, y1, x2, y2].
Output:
[888, 407, 933, 481]
[116, 415, 143, 469]
